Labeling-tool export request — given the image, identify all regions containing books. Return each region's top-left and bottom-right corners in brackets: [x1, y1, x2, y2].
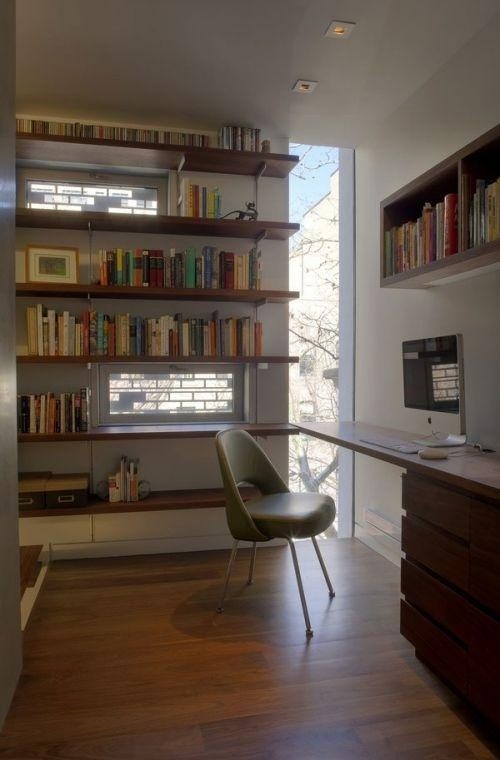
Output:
[107, 456, 141, 503]
[17, 386, 91, 433]
[383, 165, 498, 283]
[26, 303, 263, 358]
[178, 177, 222, 219]
[98, 248, 262, 290]
[14, 119, 271, 152]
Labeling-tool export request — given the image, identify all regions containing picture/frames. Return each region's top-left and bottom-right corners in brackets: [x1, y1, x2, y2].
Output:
[23, 241, 82, 286]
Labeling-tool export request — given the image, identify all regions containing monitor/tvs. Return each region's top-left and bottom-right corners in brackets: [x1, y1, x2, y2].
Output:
[402, 333, 467, 447]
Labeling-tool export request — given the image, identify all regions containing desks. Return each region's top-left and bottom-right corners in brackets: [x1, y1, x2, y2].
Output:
[299, 421, 500, 729]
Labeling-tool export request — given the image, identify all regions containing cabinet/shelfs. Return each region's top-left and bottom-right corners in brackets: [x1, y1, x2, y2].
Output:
[14, 132, 300, 517]
[379, 124, 500, 290]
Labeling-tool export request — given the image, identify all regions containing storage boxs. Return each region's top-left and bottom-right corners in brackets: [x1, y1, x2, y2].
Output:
[46, 473, 94, 508]
[18, 472, 52, 511]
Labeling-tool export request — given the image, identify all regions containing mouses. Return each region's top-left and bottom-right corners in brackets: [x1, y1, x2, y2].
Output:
[419, 449, 447, 459]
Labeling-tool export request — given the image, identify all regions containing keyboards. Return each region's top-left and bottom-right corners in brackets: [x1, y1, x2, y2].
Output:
[359, 436, 426, 454]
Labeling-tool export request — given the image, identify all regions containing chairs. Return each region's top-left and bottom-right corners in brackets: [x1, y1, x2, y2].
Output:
[214, 426, 338, 642]
